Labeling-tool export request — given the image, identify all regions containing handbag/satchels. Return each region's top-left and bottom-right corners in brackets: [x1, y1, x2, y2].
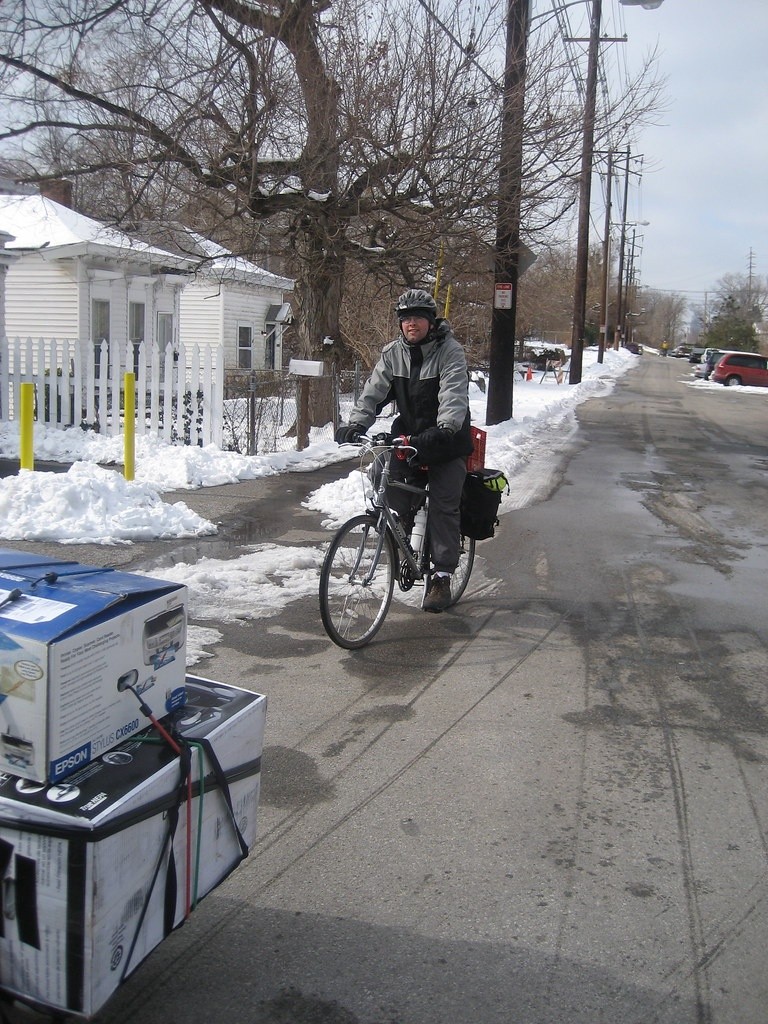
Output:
[460, 466, 510, 541]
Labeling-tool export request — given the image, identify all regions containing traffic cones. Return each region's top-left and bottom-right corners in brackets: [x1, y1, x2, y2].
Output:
[525, 366, 534, 383]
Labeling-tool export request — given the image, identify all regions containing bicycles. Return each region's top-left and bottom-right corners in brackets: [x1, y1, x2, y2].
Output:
[319, 432, 477, 651]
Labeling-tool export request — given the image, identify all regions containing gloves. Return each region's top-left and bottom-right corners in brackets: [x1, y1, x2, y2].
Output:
[334, 423, 367, 446]
[416, 427, 442, 451]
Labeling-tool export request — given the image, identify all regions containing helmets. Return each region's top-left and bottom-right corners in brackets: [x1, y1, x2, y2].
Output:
[394, 289, 437, 318]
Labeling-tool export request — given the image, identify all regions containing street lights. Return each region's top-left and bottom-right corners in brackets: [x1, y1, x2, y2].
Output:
[597, 220, 652, 362]
[484, 0, 663, 426]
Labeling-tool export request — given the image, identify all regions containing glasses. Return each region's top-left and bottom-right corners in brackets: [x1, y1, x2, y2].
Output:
[399, 316, 426, 324]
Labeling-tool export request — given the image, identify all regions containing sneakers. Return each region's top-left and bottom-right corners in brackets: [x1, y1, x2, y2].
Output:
[422, 574, 451, 611]
[398, 512, 415, 548]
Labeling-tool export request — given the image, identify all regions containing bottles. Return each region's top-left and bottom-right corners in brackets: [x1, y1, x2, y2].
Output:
[410, 506, 428, 550]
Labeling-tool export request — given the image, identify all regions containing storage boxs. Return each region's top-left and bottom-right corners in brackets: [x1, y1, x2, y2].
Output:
[0, 547, 189, 786]
[0, 676, 268, 1024]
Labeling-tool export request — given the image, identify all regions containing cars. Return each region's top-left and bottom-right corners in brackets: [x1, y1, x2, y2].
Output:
[626, 340, 644, 356]
[669, 344, 768, 387]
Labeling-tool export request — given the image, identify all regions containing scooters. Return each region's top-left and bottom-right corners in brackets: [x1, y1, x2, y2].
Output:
[659, 346, 668, 358]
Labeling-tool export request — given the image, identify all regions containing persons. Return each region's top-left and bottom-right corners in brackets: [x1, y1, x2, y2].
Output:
[335, 288, 474, 615]
[661, 340, 668, 357]
